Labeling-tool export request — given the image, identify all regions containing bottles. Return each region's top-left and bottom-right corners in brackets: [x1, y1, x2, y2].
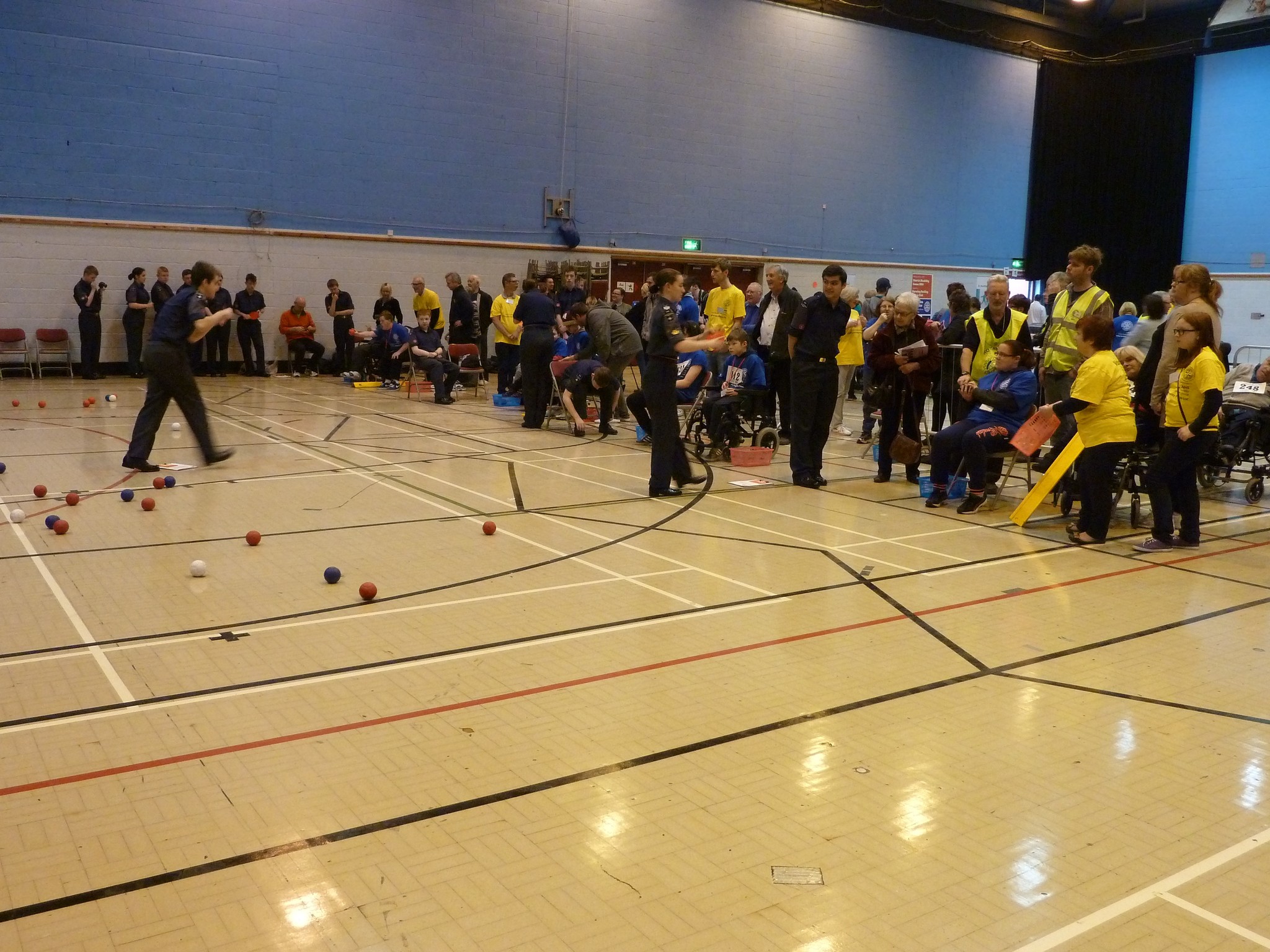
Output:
[362, 366, 367, 382]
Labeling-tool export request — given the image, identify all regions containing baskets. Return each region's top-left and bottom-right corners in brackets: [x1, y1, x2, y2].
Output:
[918, 474, 969, 499]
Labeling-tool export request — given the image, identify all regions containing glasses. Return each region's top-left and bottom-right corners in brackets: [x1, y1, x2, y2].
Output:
[1173, 328, 1194, 334]
[893, 309, 911, 316]
[411, 283, 423, 285]
[1173, 279, 1183, 285]
[994, 351, 1011, 357]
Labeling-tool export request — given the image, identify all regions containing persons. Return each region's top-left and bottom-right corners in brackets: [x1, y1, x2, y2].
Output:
[121, 260, 234, 472]
[204, 273, 232, 378]
[176, 269, 206, 376]
[122, 267, 154, 378]
[280, 244, 1270, 551]
[233, 273, 271, 377]
[151, 266, 174, 319]
[73, 265, 105, 380]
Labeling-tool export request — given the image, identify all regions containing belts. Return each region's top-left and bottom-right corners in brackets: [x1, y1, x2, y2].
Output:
[342, 315, 347, 319]
[817, 358, 826, 363]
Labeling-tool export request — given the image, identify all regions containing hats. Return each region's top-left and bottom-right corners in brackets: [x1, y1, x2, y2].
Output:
[876, 277, 890, 290]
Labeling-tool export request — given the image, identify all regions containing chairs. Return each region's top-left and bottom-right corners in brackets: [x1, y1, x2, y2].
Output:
[0, 328, 34, 380]
[546, 361, 601, 434]
[677, 371, 712, 433]
[288, 335, 320, 377]
[35, 328, 73, 379]
[947, 404, 1037, 511]
[358, 325, 489, 401]
[861, 411, 931, 459]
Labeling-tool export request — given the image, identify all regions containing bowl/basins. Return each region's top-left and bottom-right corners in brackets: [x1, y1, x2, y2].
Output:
[399, 381, 409, 386]
[353, 382, 382, 387]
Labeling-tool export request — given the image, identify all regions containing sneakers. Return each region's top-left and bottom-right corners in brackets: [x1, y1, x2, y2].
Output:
[1131, 537, 1200, 552]
[292, 369, 317, 377]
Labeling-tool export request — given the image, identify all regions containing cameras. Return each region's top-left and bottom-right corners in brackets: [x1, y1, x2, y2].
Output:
[98, 281, 107, 288]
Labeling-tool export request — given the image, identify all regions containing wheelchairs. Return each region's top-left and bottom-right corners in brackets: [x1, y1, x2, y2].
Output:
[1059, 423, 1156, 529]
[693, 382, 780, 466]
[1197, 421, 1270, 504]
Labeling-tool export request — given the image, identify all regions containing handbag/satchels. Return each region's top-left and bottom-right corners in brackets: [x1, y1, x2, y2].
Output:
[889, 432, 921, 464]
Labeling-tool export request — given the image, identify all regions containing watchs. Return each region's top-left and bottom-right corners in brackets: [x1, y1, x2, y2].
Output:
[961, 371, 970, 376]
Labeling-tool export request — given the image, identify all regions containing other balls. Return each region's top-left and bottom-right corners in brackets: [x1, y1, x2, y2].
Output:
[142, 497, 156, 511]
[105, 394, 116, 402]
[10, 509, 25, 523]
[153, 477, 165, 489]
[0, 462, 6, 474]
[33, 484, 47, 497]
[358, 582, 377, 600]
[45, 515, 61, 529]
[66, 492, 79, 506]
[38, 401, 46, 408]
[12, 399, 20, 407]
[164, 476, 176, 488]
[172, 422, 180, 430]
[482, 521, 496, 535]
[246, 530, 261, 546]
[53, 519, 69, 534]
[120, 489, 134, 502]
[190, 560, 207, 577]
[83, 396, 97, 407]
[324, 566, 341, 584]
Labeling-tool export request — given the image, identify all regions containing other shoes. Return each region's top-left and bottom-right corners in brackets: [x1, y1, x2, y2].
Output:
[613, 410, 790, 461]
[377, 376, 488, 391]
[121, 447, 232, 473]
[676, 476, 706, 488]
[435, 395, 454, 405]
[1066, 522, 1106, 544]
[792, 472, 827, 488]
[832, 424, 853, 436]
[81, 368, 271, 380]
[857, 433, 872, 443]
[1138, 509, 1182, 531]
[572, 422, 618, 436]
[1033, 460, 1050, 472]
[649, 487, 682, 498]
[874, 472, 999, 514]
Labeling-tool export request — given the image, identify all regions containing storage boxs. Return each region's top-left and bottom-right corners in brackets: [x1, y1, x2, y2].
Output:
[344, 377, 356, 382]
